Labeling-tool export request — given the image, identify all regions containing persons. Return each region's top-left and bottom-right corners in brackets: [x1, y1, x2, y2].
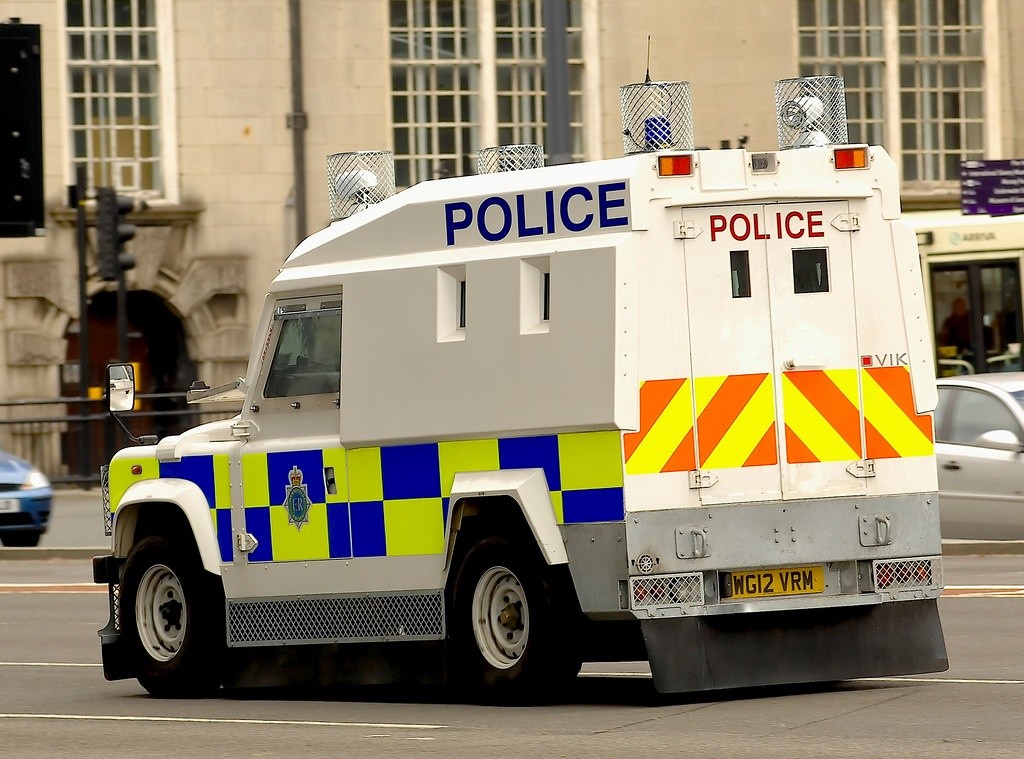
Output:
[939, 297, 972, 354]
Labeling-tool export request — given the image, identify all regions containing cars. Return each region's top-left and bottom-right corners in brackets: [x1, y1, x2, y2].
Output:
[936, 371, 1024, 538]
[0, 451, 54, 548]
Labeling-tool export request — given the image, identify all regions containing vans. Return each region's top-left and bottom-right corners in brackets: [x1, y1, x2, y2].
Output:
[897, 210, 1024, 379]
[93, 34, 948, 699]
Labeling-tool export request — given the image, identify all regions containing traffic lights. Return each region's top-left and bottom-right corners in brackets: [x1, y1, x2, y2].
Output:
[93, 185, 136, 284]
